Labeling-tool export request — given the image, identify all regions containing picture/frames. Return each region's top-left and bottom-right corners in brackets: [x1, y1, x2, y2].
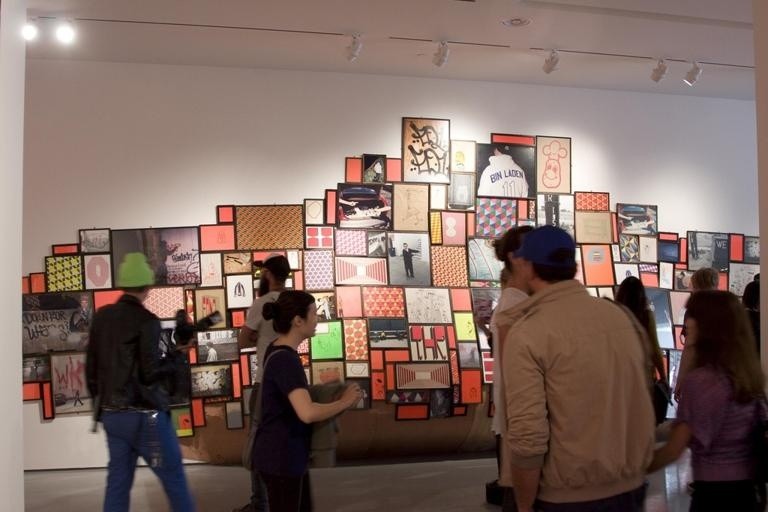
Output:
[22, 113, 760, 442]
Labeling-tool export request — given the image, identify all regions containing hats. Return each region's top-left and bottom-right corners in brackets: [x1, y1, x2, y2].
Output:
[255, 255, 290, 278]
[116, 253, 155, 288]
[513, 225, 575, 277]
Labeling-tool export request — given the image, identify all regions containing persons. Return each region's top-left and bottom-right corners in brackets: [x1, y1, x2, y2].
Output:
[251, 288, 368, 511]
[479, 146, 532, 197]
[645, 290, 768, 512]
[742, 281, 761, 355]
[205, 341, 220, 362]
[158, 308, 198, 403]
[487, 225, 666, 512]
[691, 268, 719, 292]
[403, 240, 422, 276]
[85, 252, 201, 512]
[364, 157, 383, 184]
[234, 255, 295, 434]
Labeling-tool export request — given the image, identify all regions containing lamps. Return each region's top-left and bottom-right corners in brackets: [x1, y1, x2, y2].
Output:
[340, 31, 366, 65]
[540, 47, 705, 88]
[428, 38, 452, 68]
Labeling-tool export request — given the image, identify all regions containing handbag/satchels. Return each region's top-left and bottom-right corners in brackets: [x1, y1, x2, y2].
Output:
[655, 382, 673, 423]
[242, 417, 259, 469]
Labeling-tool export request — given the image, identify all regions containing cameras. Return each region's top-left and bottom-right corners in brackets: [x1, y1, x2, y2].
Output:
[167, 310, 223, 346]
